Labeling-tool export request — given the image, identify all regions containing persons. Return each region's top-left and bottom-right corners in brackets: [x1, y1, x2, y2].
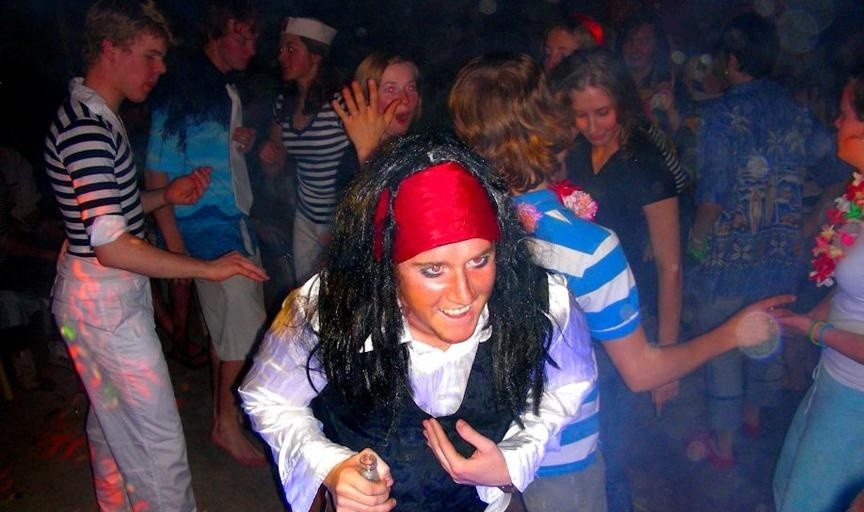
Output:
[332, 51, 796, 512]
[542, 14, 864, 512]
[237, 129, 599, 512]
[42, 2, 269, 512]
[143, 1, 423, 467]
[2, 147, 89, 423]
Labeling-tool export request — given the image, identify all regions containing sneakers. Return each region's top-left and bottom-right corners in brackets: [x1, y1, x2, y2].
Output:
[686, 437, 736, 469]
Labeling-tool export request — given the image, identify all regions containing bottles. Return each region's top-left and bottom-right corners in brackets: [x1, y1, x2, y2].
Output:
[356, 452, 381, 484]
